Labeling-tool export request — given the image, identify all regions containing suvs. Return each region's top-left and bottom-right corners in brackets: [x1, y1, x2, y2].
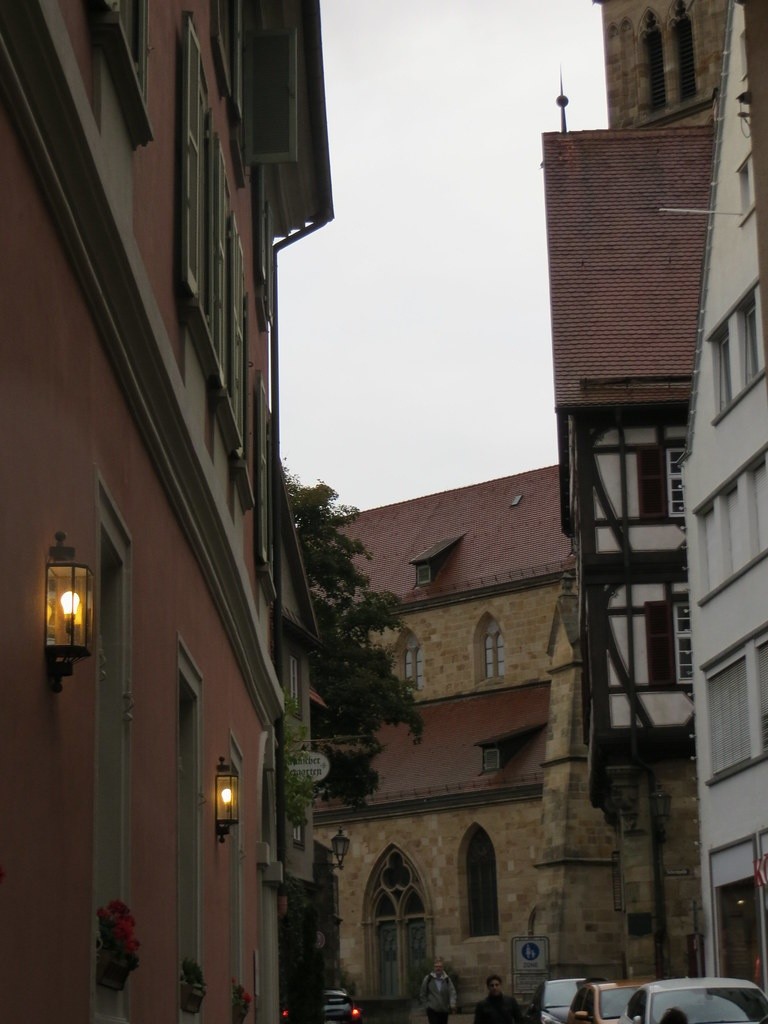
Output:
[322, 988, 362, 1024]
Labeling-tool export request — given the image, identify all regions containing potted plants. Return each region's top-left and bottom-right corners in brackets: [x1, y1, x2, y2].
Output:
[180, 957, 206, 1013]
[277, 881, 288, 916]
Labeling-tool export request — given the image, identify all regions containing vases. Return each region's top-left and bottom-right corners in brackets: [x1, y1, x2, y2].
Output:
[96, 948, 130, 991]
[232, 1005, 248, 1024]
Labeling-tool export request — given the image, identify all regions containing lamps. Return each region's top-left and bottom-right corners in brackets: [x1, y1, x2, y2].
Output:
[320, 825, 351, 870]
[215, 755, 239, 843]
[44, 530, 94, 693]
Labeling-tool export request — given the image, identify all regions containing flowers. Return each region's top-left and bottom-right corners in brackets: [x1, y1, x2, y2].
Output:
[96, 898, 141, 975]
[232, 975, 251, 1008]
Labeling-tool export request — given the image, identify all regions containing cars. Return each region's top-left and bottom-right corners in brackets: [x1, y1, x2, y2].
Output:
[527, 978, 607, 1024]
[618, 978, 768, 1024]
[567, 979, 677, 1024]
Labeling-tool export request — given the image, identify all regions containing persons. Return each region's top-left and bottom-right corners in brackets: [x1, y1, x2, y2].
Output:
[419, 958, 457, 1024]
[474, 974, 524, 1024]
